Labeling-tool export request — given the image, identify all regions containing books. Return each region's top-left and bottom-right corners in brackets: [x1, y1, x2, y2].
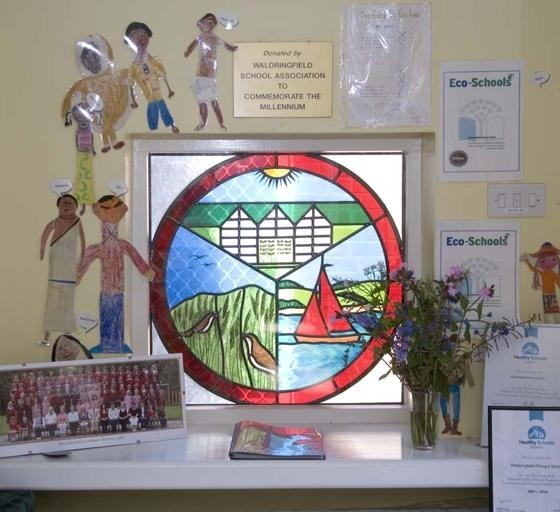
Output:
[230, 420, 325, 461]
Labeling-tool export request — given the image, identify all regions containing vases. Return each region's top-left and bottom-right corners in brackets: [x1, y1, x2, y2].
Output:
[411, 388, 439, 450]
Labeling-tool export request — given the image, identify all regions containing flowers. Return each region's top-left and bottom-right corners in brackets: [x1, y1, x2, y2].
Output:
[322, 260, 543, 450]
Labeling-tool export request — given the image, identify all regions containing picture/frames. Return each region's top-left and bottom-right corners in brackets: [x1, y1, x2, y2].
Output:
[1, 351, 190, 459]
[123, 126, 438, 435]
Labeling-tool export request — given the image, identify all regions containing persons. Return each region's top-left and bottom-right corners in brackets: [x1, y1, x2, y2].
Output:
[6, 364, 167, 442]
